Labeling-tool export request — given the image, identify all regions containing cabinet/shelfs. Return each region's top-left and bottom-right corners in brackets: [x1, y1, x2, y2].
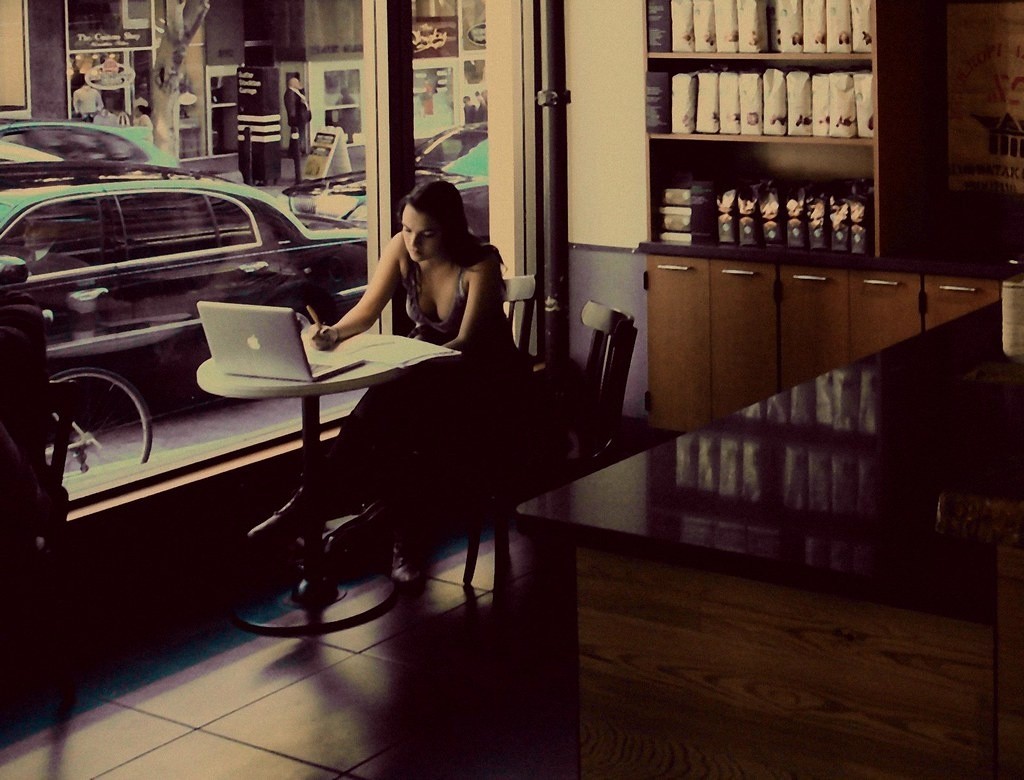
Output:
[644, 254, 778, 433]
[642, 0, 947, 257]
[923, 274, 1002, 334]
[779, 263, 923, 395]
[645, 361, 891, 578]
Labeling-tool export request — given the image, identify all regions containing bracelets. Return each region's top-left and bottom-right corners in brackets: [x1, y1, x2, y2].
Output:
[334, 327, 340, 340]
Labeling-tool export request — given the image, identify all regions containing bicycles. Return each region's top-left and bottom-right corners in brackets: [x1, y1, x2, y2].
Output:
[45, 366, 154, 487]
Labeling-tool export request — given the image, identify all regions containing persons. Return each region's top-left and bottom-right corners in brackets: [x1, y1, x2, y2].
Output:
[241, 177, 516, 592]
[71, 70, 487, 183]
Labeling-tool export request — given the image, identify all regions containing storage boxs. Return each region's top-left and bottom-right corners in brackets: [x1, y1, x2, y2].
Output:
[658, 177, 715, 233]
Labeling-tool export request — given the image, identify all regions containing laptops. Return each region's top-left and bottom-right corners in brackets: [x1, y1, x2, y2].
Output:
[197, 301, 366, 382]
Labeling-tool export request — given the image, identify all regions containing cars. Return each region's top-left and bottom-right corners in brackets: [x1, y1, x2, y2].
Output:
[0, 121, 180, 236]
[279, 121, 489, 243]
[1, 179, 368, 428]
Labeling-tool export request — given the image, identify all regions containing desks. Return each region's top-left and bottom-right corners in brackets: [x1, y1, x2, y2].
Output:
[196, 326, 411, 636]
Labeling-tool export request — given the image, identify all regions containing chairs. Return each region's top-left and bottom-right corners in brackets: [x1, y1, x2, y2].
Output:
[437, 301, 639, 584]
[457, 267, 540, 585]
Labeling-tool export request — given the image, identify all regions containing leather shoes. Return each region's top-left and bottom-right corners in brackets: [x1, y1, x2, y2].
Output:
[391, 533, 427, 595]
[246, 487, 308, 555]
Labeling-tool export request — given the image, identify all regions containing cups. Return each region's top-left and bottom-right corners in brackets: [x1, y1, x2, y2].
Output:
[1000, 260, 1024, 364]
